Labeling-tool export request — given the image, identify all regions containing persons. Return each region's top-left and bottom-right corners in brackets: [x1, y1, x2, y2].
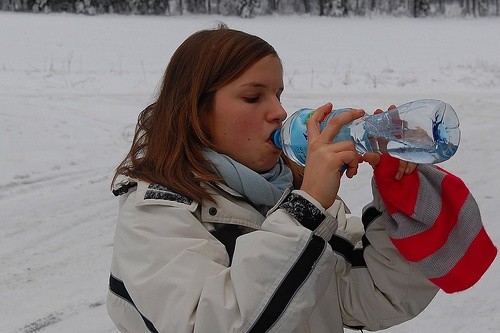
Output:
[106, 22, 440, 333]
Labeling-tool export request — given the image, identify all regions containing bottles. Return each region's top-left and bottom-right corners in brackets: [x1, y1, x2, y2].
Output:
[269, 99, 460, 164]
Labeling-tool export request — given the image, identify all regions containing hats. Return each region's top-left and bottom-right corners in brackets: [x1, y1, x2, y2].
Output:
[369, 152, 498, 293]
